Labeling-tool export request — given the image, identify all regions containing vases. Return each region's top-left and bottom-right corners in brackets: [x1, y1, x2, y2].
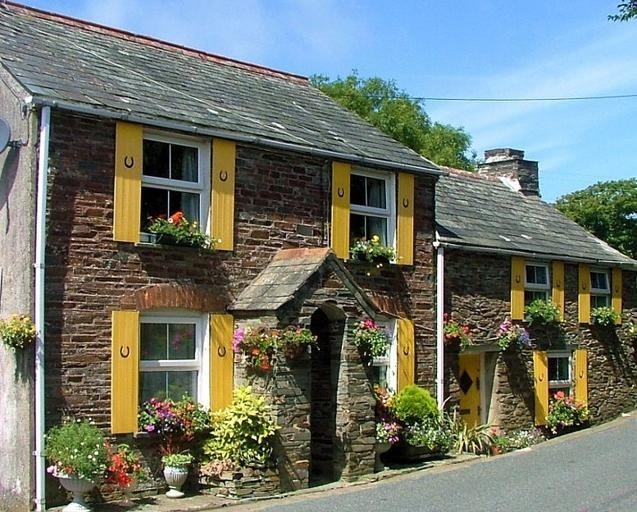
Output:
[148, 234, 199, 247]
[51, 467, 99, 512]
[118, 474, 138, 506]
[356, 251, 389, 261]
[357, 346, 372, 361]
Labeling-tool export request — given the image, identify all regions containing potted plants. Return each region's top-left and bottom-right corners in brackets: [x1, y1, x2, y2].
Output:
[161, 453, 194, 498]
[590, 305, 618, 338]
[523, 296, 559, 337]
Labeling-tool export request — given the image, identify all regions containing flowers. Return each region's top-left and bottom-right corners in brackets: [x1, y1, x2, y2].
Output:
[231, 324, 318, 369]
[353, 319, 390, 357]
[100, 444, 153, 493]
[441, 313, 470, 352]
[136, 391, 208, 450]
[496, 316, 538, 351]
[0, 313, 38, 351]
[148, 211, 222, 256]
[41, 416, 114, 484]
[370, 376, 589, 454]
[351, 235, 403, 263]
